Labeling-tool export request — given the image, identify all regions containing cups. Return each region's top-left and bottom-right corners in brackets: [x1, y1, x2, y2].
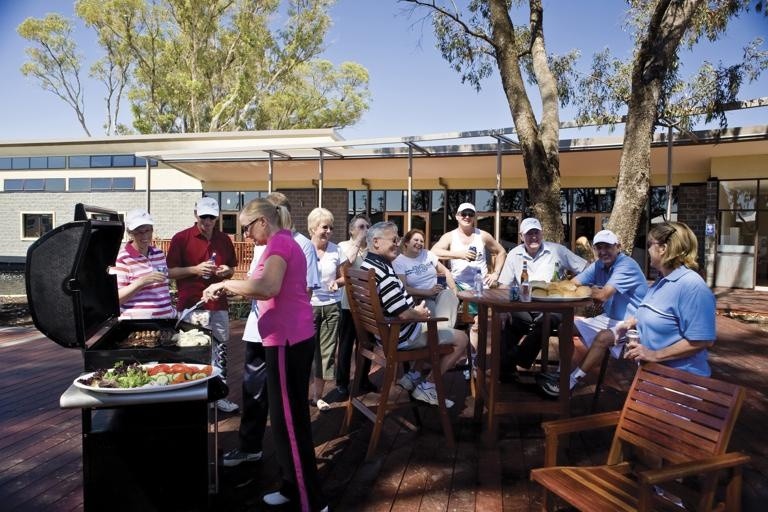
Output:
[155, 266, 167, 283]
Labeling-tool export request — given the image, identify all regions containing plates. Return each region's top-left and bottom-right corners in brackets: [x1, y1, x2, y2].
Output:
[531, 294, 591, 302]
[73, 360, 225, 395]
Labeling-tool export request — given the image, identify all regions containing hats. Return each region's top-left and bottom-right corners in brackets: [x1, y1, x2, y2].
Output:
[125, 208, 154, 234]
[520, 217, 543, 235]
[592, 228, 619, 248]
[194, 197, 220, 218]
[458, 201, 477, 213]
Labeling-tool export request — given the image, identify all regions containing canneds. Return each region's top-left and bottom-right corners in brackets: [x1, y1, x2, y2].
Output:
[469, 245, 476, 260]
[520, 283, 531, 303]
[625, 330, 641, 345]
[157, 266, 166, 274]
[509, 286, 519, 302]
[437, 274, 446, 289]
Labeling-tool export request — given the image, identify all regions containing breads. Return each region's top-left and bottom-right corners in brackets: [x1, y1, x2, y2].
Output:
[530, 279, 594, 299]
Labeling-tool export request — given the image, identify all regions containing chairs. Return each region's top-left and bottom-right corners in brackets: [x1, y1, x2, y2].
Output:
[590, 338, 628, 414]
[436, 272, 477, 400]
[531, 362, 752, 512]
[340, 264, 456, 464]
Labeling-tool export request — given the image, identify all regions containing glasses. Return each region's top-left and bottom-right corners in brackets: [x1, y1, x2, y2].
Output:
[459, 212, 476, 218]
[241, 218, 259, 231]
[199, 215, 217, 220]
[378, 236, 400, 244]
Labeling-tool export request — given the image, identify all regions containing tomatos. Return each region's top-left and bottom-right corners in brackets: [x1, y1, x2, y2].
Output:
[146, 363, 213, 375]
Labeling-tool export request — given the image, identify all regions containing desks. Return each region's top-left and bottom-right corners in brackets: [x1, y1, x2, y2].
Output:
[457, 288, 594, 449]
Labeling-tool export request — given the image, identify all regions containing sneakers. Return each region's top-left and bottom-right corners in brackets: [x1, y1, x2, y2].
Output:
[396, 368, 423, 390]
[411, 379, 456, 408]
[263, 490, 289, 506]
[462, 359, 478, 380]
[222, 446, 263, 467]
[540, 369, 571, 396]
[335, 377, 377, 394]
[209, 398, 239, 413]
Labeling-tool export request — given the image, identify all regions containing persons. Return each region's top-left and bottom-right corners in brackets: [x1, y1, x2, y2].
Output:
[167, 199, 239, 413]
[614, 221, 716, 509]
[301, 206, 468, 407]
[118, 209, 179, 322]
[202, 193, 327, 511]
[432, 202, 508, 359]
[499, 217, 589, 367]
[534, 229, 647, 397]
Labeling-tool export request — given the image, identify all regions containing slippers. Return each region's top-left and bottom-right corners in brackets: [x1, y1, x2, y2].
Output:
[310, 398, 330, 413]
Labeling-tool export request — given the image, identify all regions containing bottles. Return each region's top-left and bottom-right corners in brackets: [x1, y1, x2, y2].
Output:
[200, 252, 218, 280]
[473, 269, 484, 296]
[550, 262, 562, 282]
[509, 260, 528, 286]
